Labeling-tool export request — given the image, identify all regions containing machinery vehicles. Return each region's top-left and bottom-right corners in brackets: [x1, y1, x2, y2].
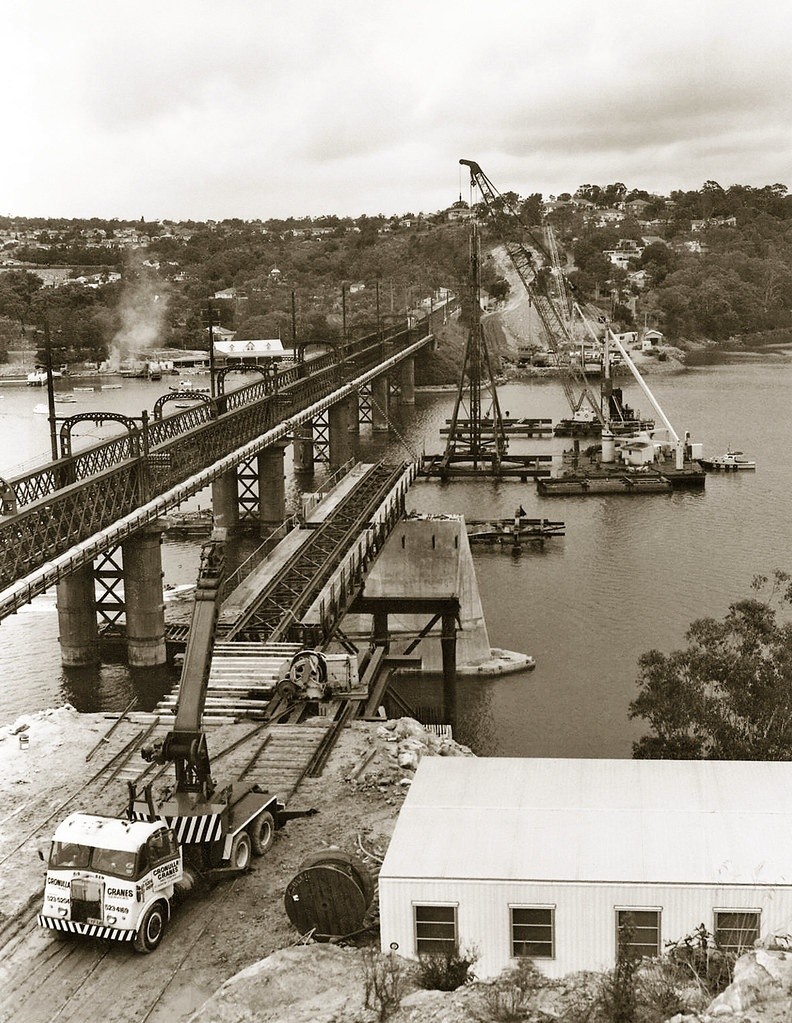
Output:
[37, 539, 318, 953]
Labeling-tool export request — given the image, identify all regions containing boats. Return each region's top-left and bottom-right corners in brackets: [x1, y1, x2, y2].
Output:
[700, 442, 756, 469]
[151, 373, 162, 380]
[175, 402, 193, 407]
[54, 392, 76, 403]
[27, 370, 48, 386]
[101, 383, 122, 390]
[554, 388, 654, 437]
[169, 381, 192, 390]
[73, 387, 94, 392]
[34, 404, 61, 415]
